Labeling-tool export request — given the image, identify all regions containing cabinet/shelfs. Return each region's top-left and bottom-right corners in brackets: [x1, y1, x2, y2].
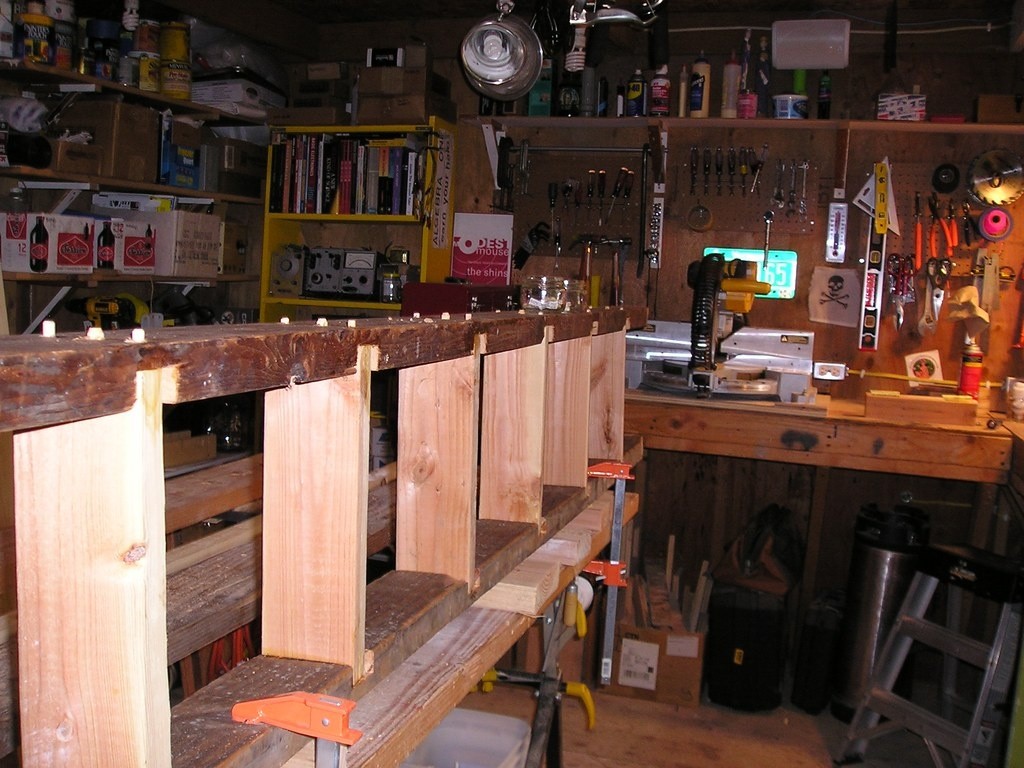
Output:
[260, 116, 457, 320]
[1, 55, 264, 335]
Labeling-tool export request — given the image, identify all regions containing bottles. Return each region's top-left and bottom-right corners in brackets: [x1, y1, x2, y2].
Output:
[84, 223, 89, 240]
[651, 66, 671, 116]
[722, 50, 743, 118]
[690, 48, 711, 117]
[627, 68, 647, 116]
[380, 272, 402, 304]
[145, 224, 152, 247]
[31, 216, 49, 271]
[98, 220, 115, 268]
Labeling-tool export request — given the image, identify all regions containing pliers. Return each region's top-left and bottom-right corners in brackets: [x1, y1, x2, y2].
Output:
[886, 253, 915, 332]
[928, 192, 953, 257]
[947, 198, 959, 246]
[961, 203, 980, 247]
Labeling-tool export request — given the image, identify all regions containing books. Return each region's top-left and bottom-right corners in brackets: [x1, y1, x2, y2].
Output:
[269, 133, 425, 217]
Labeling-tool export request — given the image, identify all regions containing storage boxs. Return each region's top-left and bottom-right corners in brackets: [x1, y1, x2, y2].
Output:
[451, 212, 515, 285]
[599, 613, 710, 705]
[973, 93, 1023, 123]
[1, 43, 456, 279]
[370, 416, 397, 472]
[875, 93, 926, 121]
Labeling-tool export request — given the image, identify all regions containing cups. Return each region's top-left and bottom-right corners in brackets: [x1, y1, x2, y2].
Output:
[521, 276, 542, 306]
[561, 279, 587, 316]
[544, 274, 565, 309]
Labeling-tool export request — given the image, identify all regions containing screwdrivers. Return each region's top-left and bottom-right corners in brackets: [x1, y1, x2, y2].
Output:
[586, 170, 595, 236]
[738, 142, 771, 197]
[727, 147, 736, 197]
[549, 184, 557, 239]
[715, 146, 723, 197]
[703, 146, 711, 196]
[597, 167, 634, 226]
[689, 145, 698, 196]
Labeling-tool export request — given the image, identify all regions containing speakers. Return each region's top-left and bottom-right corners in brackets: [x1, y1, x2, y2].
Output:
[772, 19, 850, 70]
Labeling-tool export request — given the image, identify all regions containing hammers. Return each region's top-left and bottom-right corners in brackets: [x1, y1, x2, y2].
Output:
[597, 237, 632, 305]
[576, 235, 607, 280]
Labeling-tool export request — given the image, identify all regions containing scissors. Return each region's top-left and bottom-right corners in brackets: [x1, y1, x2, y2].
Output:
[926, 258, 952, 322]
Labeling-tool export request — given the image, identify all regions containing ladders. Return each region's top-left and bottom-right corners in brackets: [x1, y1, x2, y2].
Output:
[832, 542, 1024, 768]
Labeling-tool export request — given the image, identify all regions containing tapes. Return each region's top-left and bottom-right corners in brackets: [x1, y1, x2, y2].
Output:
[977, 207, 1014, 242]
[685, 204, 714, 233]
